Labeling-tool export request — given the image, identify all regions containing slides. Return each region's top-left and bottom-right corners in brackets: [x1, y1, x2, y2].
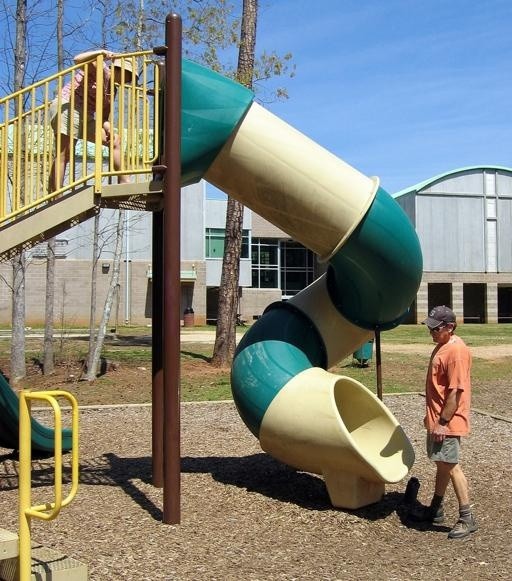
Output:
[157, 57, 422, 509]
[0, 374, 73, 452]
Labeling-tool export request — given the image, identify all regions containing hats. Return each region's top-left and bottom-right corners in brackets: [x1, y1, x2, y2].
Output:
[109, 57, 139, 81]
[421, 305, 456, 329]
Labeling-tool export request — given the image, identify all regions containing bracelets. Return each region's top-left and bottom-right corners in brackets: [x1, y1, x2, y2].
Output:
[437, 417, 450, 428]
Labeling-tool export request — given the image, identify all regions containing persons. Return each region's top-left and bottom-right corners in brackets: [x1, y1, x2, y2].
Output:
[45, 48, 138, 204]
[407, 302, 480, 541]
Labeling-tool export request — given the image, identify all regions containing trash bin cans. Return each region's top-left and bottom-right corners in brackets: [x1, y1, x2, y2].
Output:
[353, 337, 374, 367]
[184, 307, 194, 327]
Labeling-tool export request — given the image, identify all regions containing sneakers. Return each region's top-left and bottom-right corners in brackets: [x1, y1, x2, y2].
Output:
[412, 503, 445, 522]
[448, 515, 478, 538]
